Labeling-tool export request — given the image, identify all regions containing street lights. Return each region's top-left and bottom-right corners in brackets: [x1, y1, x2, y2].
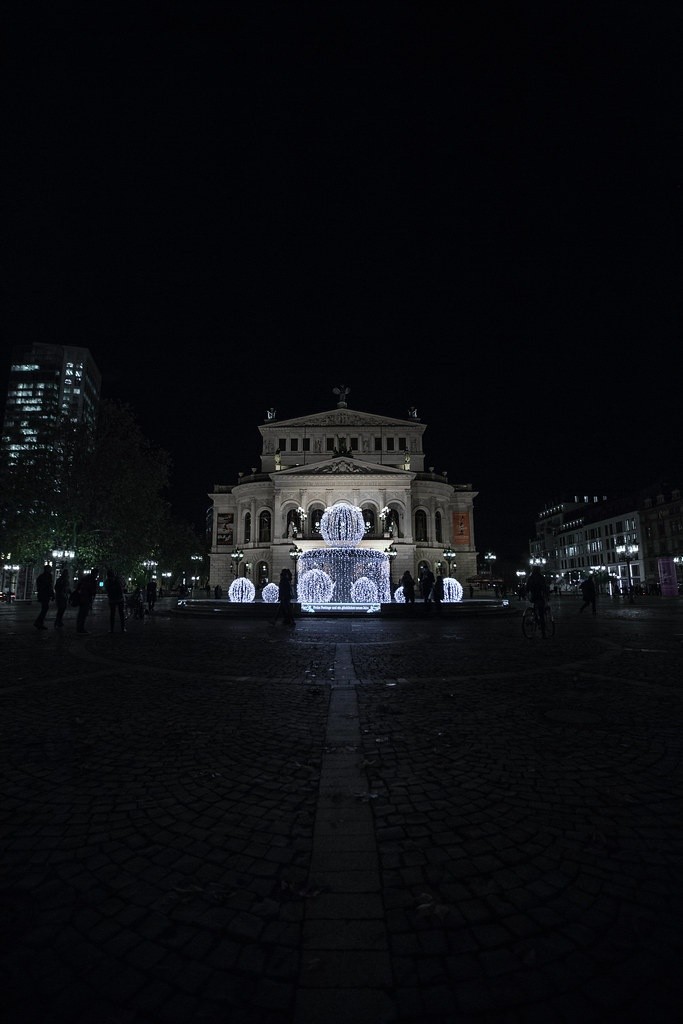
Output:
[484, 550, 497, 583]
[289, 545, 303, 584]
[442, 546, 457, 578]
[190, 552, 203, 589]
[516, 568, 527, 590]
[230, 546, 244, 579]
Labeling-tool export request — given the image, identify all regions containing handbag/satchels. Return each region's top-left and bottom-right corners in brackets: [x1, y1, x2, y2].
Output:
[69, 591, 79, 606]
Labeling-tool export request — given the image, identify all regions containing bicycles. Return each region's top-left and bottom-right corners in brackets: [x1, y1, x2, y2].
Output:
[522, 589, 557, 639]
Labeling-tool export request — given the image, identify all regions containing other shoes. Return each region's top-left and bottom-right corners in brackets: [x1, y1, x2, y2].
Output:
[55, 623, 63, 627]
[108, 630, 113, 633]
[34, 624, 47, 630]
[122, 628, 127, 631]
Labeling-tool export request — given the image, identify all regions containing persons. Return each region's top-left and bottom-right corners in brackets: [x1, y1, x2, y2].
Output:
[104, 569, 127, 633]
[494, 583, 500, 599]
[554, 586, 562, 599]
[190, 582, 199, 589]
[402, 570, 415, 603]
[578, 575, 598, 615]
[205, 582, 211, 598]
[432, 575, 444, 612]
[527, 566, 550, 633]
[638, 583, 662, 597]
[169, 586, 174, 590]
[174, 585, 190, 599]
[516, 583, 526, 601]
[75, 568, 99, 635]
[132, 587, 144, 621]
[419, 566, 435, 604]
[614, 583, 621, 599]
[623, 583, 637, 600]
[33, 565, 55, 630]
[501, 583, 508, 600]
[54, 569, 71, 627]
[147, 582, 158, 611]
[159, 587, 164, 597]
[469, 584, 474, 598]
[272, 572, 295, 626]
[214, 585, 223, 599]
